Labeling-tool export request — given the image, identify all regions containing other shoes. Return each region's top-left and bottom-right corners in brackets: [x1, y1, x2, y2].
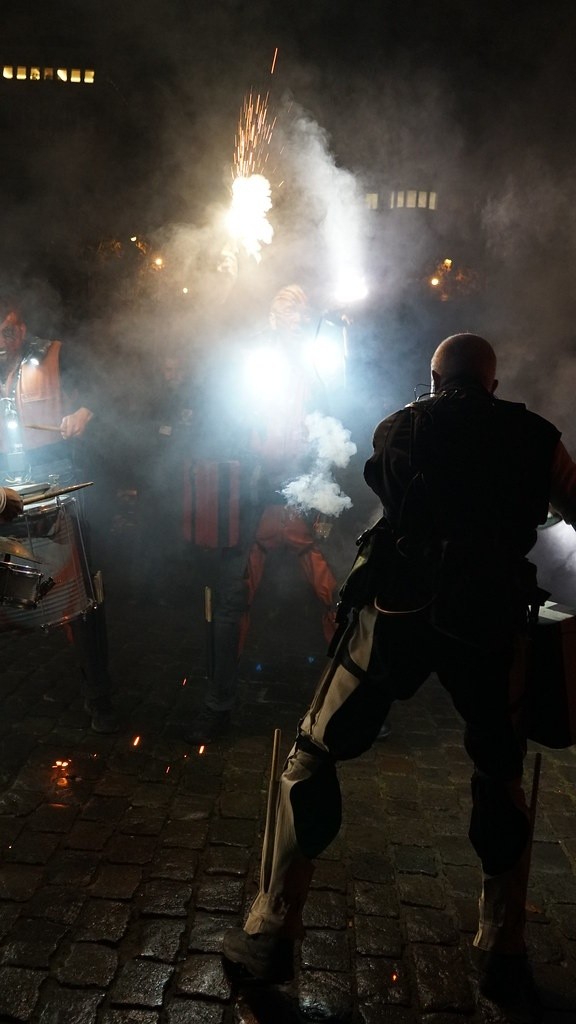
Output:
[187, 707, 231, 744]
[477, 949, 532, 1004]
[83, 696, 119, 734]
[220, 927, 296, 981]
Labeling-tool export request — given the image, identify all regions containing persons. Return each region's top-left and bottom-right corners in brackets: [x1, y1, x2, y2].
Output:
[221, 333, 576, 984]
[170, 282, 396, 747]
[0, 304, 105, 486]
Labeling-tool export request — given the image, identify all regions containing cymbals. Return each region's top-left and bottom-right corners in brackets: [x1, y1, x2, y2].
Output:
[0, 535, 42, 564]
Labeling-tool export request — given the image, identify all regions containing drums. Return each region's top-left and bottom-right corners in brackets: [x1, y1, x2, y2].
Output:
[0, 561, 45, 610]
[0, 494, 99, 636]
